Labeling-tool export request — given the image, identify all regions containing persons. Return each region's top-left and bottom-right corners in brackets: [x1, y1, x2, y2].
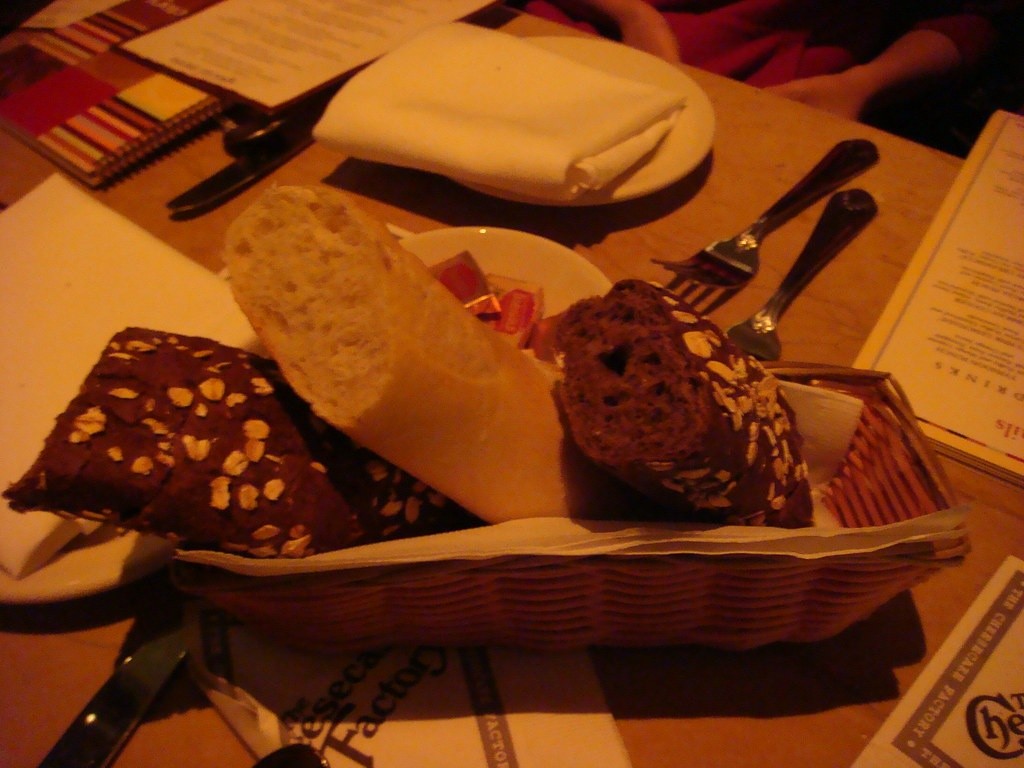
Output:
[557, 1, 997, 126]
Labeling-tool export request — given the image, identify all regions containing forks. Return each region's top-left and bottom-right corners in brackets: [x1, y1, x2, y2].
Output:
[651, 138, 880, 289]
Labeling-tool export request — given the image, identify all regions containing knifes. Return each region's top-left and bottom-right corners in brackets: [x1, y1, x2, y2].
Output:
[165, 82, 343, 214]
[36, 635, 187, 768]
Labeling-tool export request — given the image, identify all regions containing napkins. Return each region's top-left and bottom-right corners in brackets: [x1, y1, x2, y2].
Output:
[0, 173, 262, 581]
[312, 21, 687, 202]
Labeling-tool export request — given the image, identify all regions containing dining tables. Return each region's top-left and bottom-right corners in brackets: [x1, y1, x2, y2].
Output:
[0, 0, 1024, 768]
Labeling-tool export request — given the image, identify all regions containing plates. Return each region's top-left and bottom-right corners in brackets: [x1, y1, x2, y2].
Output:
[449, 36, 715, 206]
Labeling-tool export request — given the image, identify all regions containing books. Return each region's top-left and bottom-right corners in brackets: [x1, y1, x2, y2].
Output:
[1, 46, 230, 190]
[851, 110, 1023, 484]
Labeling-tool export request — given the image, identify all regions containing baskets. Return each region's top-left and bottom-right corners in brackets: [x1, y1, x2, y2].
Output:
[159, 358, 974, 652]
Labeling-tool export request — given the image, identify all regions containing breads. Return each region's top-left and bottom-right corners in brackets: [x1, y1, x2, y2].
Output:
[0, 188, 814, 557]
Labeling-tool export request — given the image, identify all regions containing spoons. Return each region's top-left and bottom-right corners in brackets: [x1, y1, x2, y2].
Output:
[726, 190, 878, 360]
